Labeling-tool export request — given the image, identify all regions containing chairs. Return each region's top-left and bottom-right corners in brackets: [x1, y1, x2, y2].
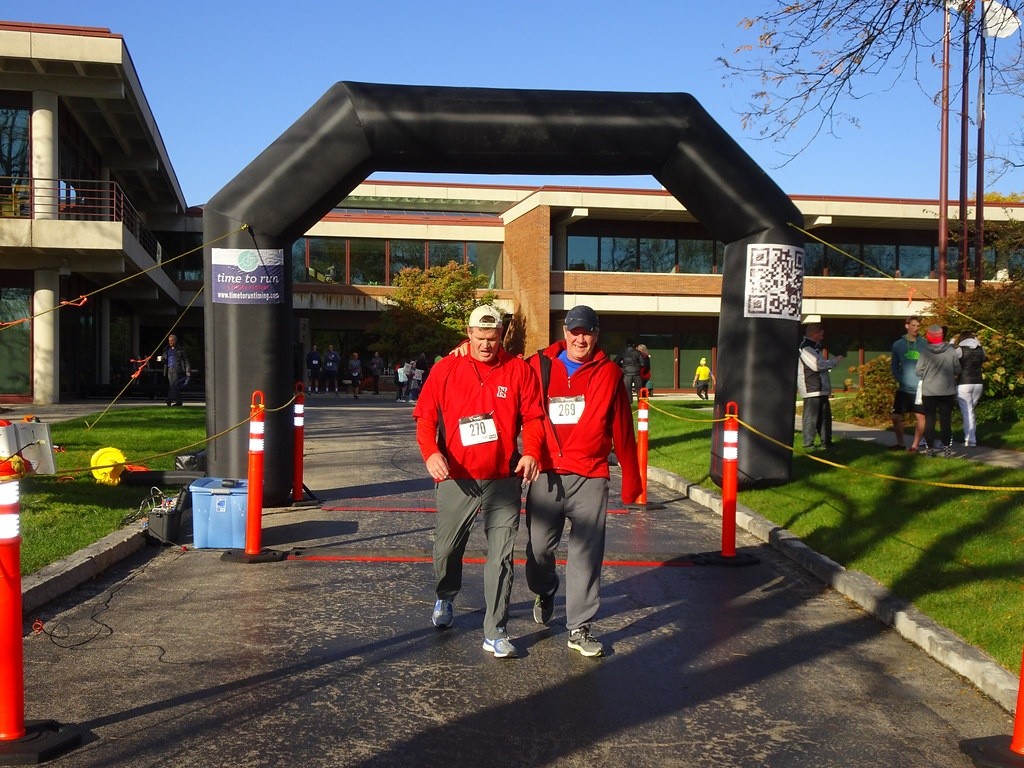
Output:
[12, 185, 30, 217]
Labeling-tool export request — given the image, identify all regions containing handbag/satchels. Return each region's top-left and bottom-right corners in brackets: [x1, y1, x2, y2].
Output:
[914, 378, 923, 406]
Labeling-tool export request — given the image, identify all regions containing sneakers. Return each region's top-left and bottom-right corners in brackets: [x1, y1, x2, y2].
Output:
[533, 578, 559, 624]
[568, 629, 604, 657]
[483, 635, 518, 658]
[432, 597, 454, 628]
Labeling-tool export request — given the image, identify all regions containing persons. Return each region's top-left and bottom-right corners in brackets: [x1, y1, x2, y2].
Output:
[891, 316, 932, 453]
[915, 324, 962, 457]
[523, 306, 643, 658]
[693, 358, 716, 400]
[412, 304, 545, 658]
[952, 331, 988, 448]
[613, 338, 651, 404]
[161, 335, 192, 407]
[394, 351, 443, 403]
[306, 344, 323, 395]
[349, 353, 363, 399]
[323, 344, 340, 394]
[796, 322, 844, 449]
[370, 351, 384, 395]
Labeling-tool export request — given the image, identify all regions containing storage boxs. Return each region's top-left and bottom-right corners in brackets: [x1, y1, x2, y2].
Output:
[189, 478, 248, 551]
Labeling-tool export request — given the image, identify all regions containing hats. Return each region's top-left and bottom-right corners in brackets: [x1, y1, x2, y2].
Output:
[700, 357, 707, 364]
[564, 306, 600, 332]
[625, 339, 634, 346]
[925, 323, 943, 343]
[469, 305, 503, 328]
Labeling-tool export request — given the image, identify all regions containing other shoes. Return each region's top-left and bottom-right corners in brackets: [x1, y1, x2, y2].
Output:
[962, 441, 976, 447]
[888, 445, 906, 451]
[920, 447, 933, 456]
[908, 447, 919, 452]
[943, 445, 952, 458]
